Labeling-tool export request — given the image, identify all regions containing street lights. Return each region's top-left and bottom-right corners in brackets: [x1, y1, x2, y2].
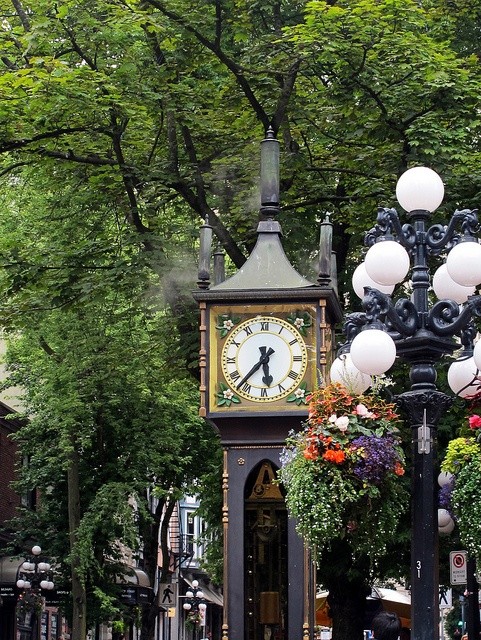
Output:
[328, 164, 481, 640]
[183, 580, 207, 640]
[16, 545, 55, 639]
[434, 465, 481, 640]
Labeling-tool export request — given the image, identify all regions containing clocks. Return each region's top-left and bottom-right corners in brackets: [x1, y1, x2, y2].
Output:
[195, 122, 342, 639]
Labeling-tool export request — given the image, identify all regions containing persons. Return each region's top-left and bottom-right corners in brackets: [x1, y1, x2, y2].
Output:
[399, 626, 410, 640]
[369, 611, 401, 640]
